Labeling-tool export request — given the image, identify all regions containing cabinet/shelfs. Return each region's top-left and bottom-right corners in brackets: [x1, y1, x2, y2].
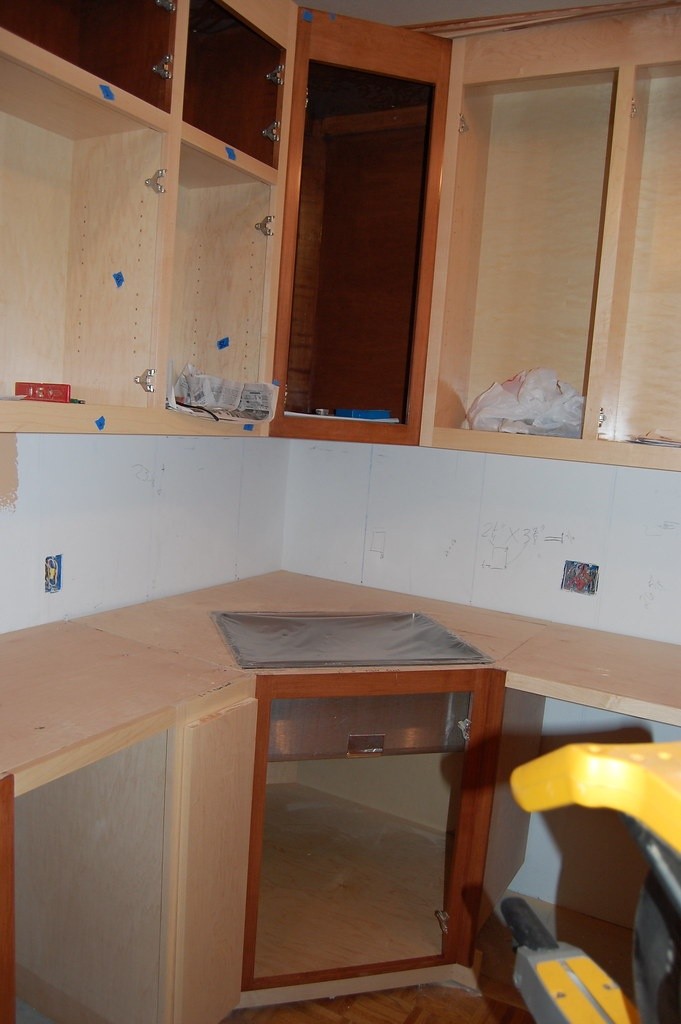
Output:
[244, 675, 516, 1004]
[425, 7, 679, 471]
[1, 697, 266, 1023]
[270, 10, 453, 446]
[1, 2, 300, 439]
